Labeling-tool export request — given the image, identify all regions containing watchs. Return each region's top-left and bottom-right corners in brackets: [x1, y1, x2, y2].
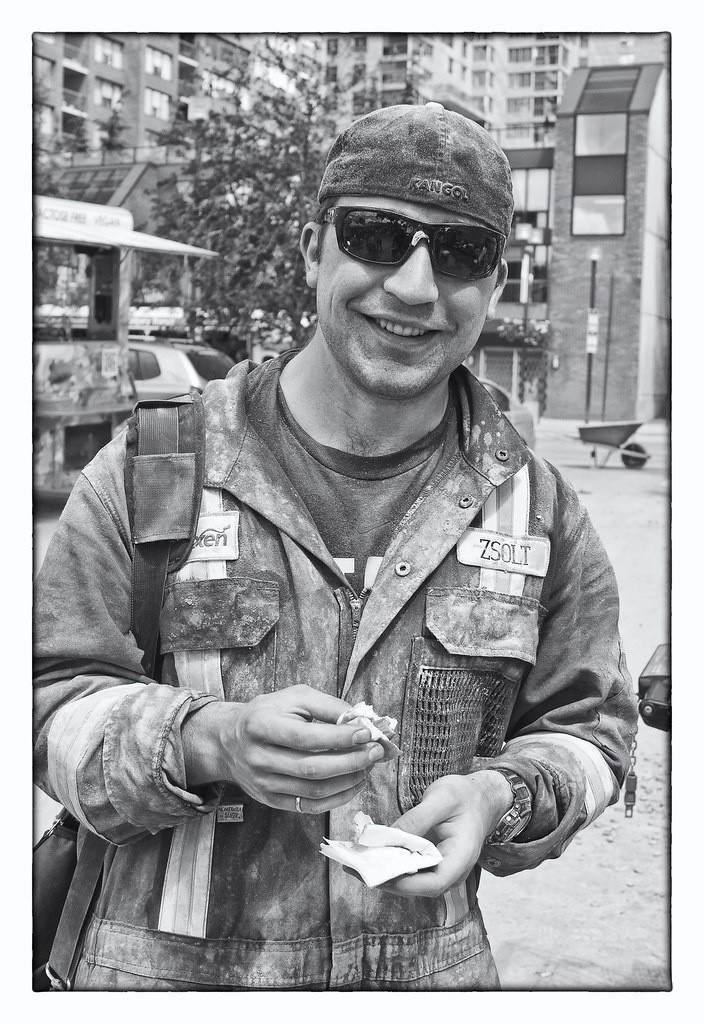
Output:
[484, 769, 532, 846]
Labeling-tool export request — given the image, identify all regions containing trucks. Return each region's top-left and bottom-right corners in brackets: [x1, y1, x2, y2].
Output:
[33, 194, 221, 503]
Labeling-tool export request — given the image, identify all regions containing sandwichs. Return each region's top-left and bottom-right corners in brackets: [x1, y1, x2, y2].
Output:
[337, 702, 403, 763]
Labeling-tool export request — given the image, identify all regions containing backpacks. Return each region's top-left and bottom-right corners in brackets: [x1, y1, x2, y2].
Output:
[32, 392, 207, 992]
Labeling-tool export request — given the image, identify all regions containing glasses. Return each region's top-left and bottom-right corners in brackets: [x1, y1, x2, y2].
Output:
[321, 205, 508, 283]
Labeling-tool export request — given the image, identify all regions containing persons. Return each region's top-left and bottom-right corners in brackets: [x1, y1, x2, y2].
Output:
[33, 104, 639, 991]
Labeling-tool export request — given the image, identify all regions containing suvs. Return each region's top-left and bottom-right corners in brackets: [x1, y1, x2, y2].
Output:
[129, 331, 235, 409]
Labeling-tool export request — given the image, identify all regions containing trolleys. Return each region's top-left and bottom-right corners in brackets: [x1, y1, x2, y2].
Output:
[563, 422, 650, 469]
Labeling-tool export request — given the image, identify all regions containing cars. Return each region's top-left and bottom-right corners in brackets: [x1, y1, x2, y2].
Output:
[477, 376, 536, 450]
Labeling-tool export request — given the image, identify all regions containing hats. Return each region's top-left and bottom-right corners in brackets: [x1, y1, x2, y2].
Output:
[317, 102, 515, 239]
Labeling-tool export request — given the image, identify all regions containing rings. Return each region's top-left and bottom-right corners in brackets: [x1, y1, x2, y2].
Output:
[296, 797, 304, 813]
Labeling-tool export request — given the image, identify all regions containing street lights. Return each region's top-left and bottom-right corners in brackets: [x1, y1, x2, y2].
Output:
[584, 246, 604, 425]
